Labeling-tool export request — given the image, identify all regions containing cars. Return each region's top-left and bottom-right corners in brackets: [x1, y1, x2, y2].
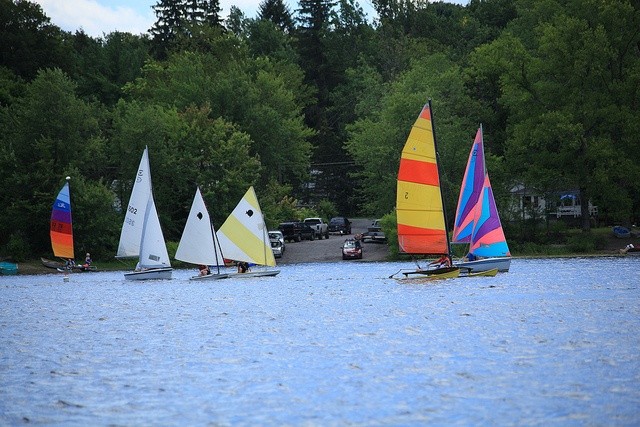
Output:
[268, 231, 285, 250]
[328, 217, 352, 234]
[371, 219, 385, 228]
[340, 239, 364, 259]
[270, 238, 283, 258]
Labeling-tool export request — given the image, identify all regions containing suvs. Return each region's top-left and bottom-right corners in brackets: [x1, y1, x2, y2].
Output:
[278, 222, 315, 243]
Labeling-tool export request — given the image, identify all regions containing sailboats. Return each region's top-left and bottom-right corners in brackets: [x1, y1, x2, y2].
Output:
[124, 197, 173, 280]
[453, 122, 488, 264]
[173, 187, 230, 282]
[398, 95, 498, 281]
[115, 145, 155, 260]
[215, 185, 281, 279]
[50, 174, 81, 274]
[453, 172, 512, 273]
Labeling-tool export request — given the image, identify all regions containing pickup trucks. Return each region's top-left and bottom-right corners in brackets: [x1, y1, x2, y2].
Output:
[303, 218, 330, 240]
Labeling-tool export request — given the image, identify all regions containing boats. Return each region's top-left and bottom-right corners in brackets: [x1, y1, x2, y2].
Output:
[41, 255, 81, 269]
